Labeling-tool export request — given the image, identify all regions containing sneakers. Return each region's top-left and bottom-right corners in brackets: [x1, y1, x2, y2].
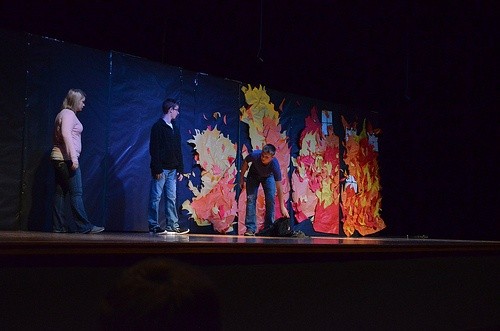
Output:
[53, 226, 71, 233]
[149, 226, 166, 235]
[89, 226, 104, 234]
[244, 231, 255, 236]
[166, 228, 190, 235]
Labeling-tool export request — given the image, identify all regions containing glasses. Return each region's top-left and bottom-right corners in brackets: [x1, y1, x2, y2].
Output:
[172, 108, 179, 111]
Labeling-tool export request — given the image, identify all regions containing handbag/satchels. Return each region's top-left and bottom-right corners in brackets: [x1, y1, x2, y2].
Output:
[268, 216, 294, 237]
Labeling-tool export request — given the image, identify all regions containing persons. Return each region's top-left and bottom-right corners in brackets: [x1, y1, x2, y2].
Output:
[240, 144, 290, 236]
[49, 89, 105, 234]
[147, 98, 190, 235]
